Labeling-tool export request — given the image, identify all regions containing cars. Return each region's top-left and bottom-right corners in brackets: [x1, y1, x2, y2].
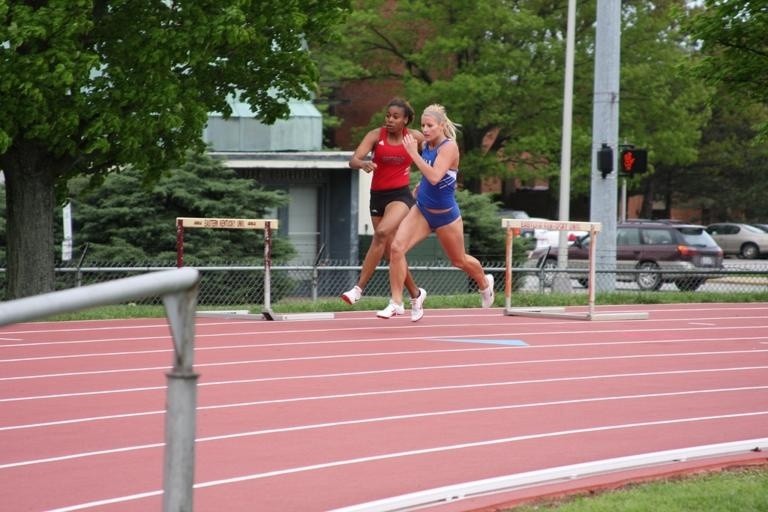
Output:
[524, 219, 768, 291]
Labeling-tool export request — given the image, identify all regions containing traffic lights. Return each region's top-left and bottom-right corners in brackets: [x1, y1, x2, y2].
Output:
[621, 149, 647, 173]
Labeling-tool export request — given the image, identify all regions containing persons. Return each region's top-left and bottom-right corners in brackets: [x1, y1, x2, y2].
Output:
[374, 104, 495, 321]
[340, 96, 427, 322]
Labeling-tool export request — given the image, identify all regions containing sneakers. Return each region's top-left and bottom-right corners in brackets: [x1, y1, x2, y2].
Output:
[479, 274, 496, 308]
[409, 287, 428, 322]
[375, 301, 405, 320]
[341, 286, 363, 304]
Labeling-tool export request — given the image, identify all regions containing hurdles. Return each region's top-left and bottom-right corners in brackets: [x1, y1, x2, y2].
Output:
[501, 218, 648, 320]
[175, 217, 334, 321]
